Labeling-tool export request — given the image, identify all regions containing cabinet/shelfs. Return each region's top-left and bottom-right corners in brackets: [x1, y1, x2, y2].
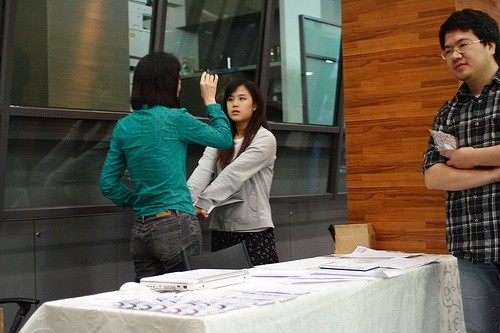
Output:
[180, 61, 282, 120]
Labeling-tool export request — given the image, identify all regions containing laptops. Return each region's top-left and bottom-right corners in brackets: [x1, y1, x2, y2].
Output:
[140, 268, 252, 291]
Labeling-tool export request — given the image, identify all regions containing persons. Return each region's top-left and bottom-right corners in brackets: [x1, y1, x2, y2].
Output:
[186, 81, 277, 268]
[421, 8, 500, 333]
[100, 52, 233, 285]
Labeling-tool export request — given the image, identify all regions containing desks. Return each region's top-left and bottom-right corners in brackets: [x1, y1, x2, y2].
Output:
[17, 254, 467, 333]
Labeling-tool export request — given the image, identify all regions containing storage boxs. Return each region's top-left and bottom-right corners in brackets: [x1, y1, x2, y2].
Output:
[334, 222, 377, 253]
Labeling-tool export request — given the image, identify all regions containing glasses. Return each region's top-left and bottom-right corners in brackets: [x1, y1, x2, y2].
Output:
[440, 39, 483, 59]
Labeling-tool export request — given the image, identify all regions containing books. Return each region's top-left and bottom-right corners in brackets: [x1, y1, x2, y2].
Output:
[320, 246, 421, 272]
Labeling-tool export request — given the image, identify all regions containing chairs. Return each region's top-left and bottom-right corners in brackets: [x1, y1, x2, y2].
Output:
[182, 240, 252, 270]
